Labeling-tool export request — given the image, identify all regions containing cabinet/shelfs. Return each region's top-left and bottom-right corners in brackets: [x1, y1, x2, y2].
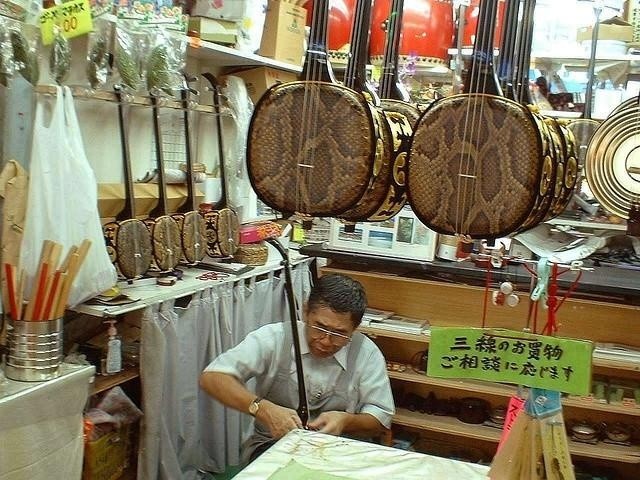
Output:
[299, 240, 640, 479]
[61, 250, 311, 396]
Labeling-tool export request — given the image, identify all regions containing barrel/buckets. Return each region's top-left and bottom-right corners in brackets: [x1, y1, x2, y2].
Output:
[3, 311, 65, 382]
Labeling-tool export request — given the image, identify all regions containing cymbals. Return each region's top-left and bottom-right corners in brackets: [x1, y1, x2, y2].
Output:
[583, 95, 640, 223]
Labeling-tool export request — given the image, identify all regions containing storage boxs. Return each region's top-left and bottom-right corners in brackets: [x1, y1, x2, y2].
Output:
[257, 0, 309, 66]
[577, 14, 635, 44]
[219, 65, 297, 110]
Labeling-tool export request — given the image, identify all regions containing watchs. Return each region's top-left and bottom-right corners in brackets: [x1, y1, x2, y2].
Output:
[248, 396, 264, 419]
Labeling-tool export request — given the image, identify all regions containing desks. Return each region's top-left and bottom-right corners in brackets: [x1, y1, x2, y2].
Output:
[225, 427, 493, 480]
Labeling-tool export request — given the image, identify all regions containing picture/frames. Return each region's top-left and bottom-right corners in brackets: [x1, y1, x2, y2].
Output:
[327, 206, 439, 263]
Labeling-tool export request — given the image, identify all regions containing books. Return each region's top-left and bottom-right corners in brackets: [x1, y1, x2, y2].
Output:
[361, 305, 431, 338]
[594, 339, 640, 365]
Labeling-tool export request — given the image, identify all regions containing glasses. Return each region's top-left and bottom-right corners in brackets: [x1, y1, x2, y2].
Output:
[303, 315, 354, 348]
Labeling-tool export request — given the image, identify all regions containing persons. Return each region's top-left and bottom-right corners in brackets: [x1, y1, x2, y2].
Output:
[198, 272, 396, 466]
